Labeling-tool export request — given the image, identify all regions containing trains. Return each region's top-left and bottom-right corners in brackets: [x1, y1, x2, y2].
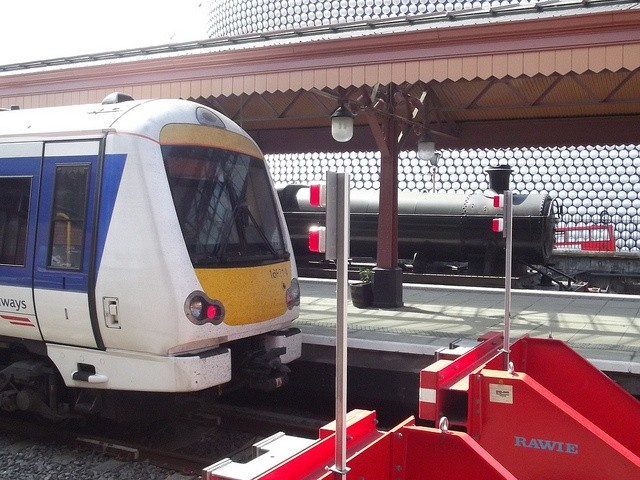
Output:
[273, 185, 558, 276]
[0, 92, 301, 427]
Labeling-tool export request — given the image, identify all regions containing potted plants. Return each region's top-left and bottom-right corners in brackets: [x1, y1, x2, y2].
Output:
[349, 267, 374, 308]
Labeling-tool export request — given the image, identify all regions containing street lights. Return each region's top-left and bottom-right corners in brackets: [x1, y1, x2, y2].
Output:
[330, 80, 435, 307]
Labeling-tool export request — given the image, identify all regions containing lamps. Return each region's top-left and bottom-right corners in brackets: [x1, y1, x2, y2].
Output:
[416, 128, 435, 162]
[328, 89, 356, 143]
